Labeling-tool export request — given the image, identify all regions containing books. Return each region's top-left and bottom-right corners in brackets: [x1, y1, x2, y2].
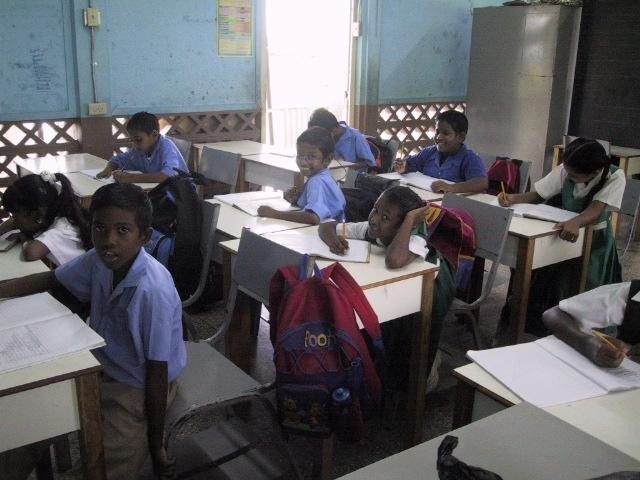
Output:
[377, 171, 455, 191]
[263, 233, 372, 264]
[0, 229, 21, 252]
[80, 170, 159, 189]
[0, 290, 108, 376]
[492, 196, 581, 223]
[212, 190, 302, 216]
[464, 334, 640, 410]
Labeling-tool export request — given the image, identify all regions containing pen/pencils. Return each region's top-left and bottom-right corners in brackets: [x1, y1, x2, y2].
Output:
[342, 218, 346, 238]
[400, 144, 403, 159]
[500, 181, 507, 201]
[592, 331, 628, 359]
[122, 167, 124, 172]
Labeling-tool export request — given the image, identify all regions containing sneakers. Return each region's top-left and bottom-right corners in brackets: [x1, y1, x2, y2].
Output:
[425, 349, 443, 394]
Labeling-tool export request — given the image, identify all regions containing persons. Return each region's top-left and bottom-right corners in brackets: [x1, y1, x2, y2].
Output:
[0, 171, 94, 270]
[540, 277, 640, 366]
[98, 112, 192, 185]
[0, 182, 188, 480]
[390, 109, 491, 193]
[315, 185, 456, 396]
[307, 108, 377, 171]
[495, 136, 629, 338]
[257, 127, 348, 226]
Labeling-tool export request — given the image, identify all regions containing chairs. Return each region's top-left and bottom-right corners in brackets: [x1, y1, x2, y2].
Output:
[350, 172, 402, 228]
[422, 194, 517, 355]
[136, 228, 315, 476]
[601, 176, 639, 263]
[157, 133, 192, 173]
[196, 142, 250, 194]
[472, 148, 529, 194]
[365, 133, 400, 171]
[178, 199, 227, 308]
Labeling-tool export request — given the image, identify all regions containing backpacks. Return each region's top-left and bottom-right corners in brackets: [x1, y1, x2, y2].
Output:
[268, 253, 388, 438]
[366, 137, 392, 174]
[417, 202, 477, 303]
[142, 174, 204, 300]
[486, 156, 531, 196]
[340, 186, 382, 224]
